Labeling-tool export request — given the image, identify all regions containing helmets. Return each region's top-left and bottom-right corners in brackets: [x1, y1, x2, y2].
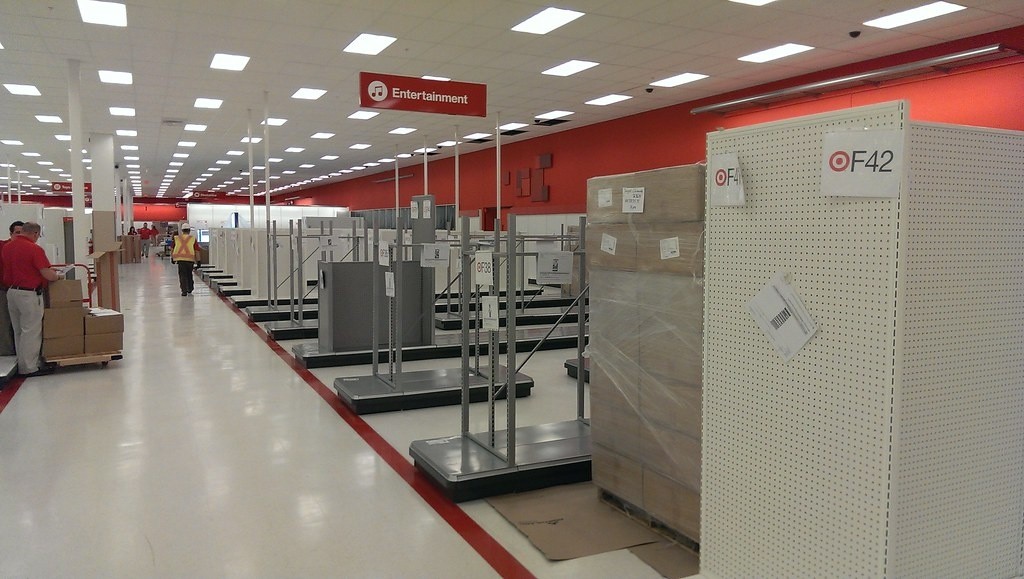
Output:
[181, 223, 190, 230]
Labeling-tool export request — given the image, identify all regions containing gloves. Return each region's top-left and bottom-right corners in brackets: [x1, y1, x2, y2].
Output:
[171, 258, 175, 264]
[197, 261, 201, 268]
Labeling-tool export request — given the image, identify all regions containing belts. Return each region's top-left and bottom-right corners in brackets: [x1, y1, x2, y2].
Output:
[11, 286, 37, 291]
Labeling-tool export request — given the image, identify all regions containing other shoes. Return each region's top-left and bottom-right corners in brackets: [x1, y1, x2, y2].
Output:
[19, 368, 49, 377]
[182, 292, 187, 296]
[188, 288, 194, 293]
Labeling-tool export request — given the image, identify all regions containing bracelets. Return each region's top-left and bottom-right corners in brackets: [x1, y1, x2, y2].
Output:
[57, 275, 60, 280]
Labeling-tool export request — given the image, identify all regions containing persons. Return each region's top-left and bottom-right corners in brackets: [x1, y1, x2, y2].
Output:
[170, 223, 201, 296]
[0, 221, 25, 357]
[2, 220, 65, 378]
[151, 225, 159, 247]
[139, 223, 152, 258]
[128, 227, 137, 235]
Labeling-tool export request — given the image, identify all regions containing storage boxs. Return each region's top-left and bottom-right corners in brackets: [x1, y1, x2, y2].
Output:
[583, 164, 708, 545]
[85, 332, 124, 355]
[84, 306, 125, 334]
[41, 336, 86, 359]
[44, 279, 83, 307]
[43, 309, 85, 339]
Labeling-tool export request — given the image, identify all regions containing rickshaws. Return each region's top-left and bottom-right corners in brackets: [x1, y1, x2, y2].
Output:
[38, 263, 122, 374]
